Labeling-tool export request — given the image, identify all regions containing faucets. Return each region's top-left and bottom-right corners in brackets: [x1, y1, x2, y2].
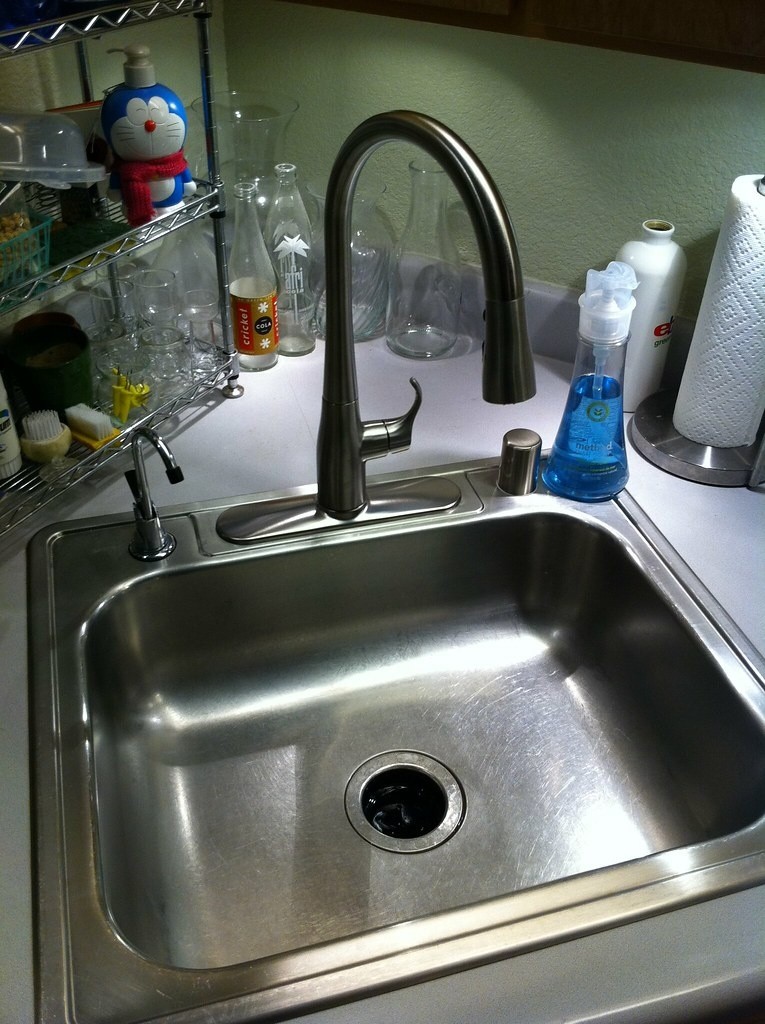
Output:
[313, 108, 537, 521]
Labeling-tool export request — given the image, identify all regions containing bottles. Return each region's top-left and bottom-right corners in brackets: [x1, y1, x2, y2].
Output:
[305, 173, 398, 342]
[262, 160, 315, 356]
[228, 180, 282, 373]
[616, 218, 690, 413]
[190, 89, 319, 243]
[384, 159, 463, 360]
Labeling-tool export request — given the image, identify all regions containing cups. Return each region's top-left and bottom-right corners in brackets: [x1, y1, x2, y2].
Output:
[10, 311, 94, 413]
[84, 259, 221, 424]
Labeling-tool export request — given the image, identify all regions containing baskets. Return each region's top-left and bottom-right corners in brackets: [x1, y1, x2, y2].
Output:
[0, 199, 54, 313]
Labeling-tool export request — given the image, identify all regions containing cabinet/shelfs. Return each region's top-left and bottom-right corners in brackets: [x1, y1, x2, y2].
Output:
[0, 0, 219, 536]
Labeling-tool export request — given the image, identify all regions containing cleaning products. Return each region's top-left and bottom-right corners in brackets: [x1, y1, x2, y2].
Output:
[533, 260, 640, 501]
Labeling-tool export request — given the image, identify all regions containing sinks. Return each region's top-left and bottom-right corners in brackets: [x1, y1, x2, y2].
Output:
[22, 443, 765, 1024]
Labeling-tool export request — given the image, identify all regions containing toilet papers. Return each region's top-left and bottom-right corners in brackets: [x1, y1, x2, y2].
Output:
[667, 172, 765, 448]
